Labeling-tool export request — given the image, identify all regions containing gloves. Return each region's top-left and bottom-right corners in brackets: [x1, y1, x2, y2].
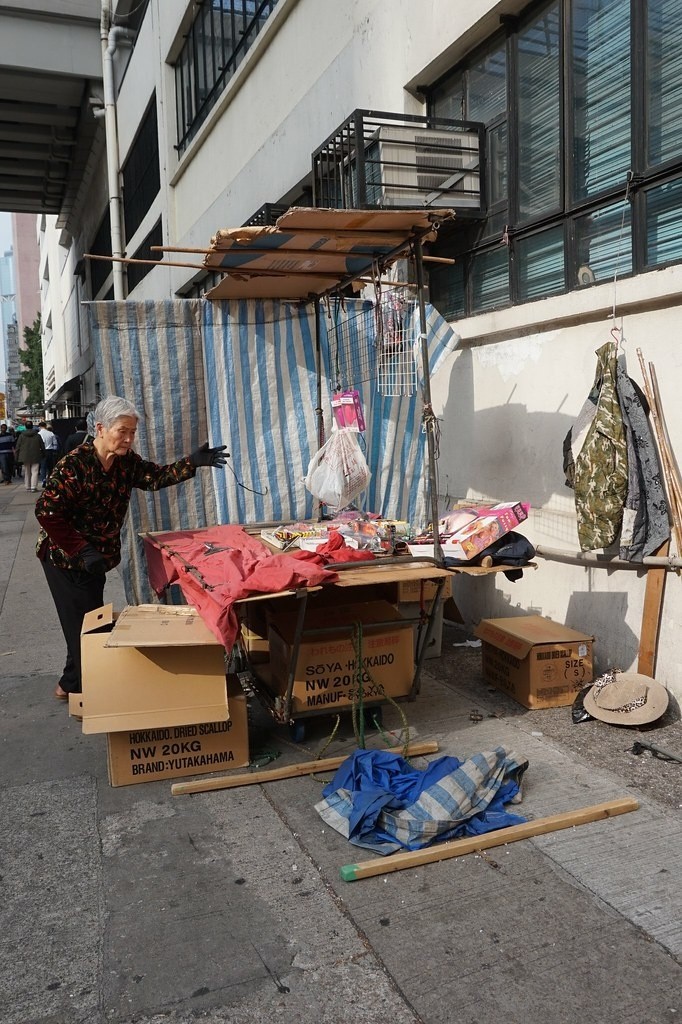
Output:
[78, 543, 108, 574]
[189, 442, 230, 469]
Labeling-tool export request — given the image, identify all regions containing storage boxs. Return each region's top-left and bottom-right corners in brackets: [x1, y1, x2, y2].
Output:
[473, 614, 595, 710]
[68, 603, 248, 788]
[268, 600, 417, 714]
[386, 576, 453, 603]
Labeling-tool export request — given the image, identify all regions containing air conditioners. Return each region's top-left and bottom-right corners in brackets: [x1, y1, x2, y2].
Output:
[339, 125, 482, 208]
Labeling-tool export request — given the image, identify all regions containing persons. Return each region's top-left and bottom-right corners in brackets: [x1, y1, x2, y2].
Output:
[0, 420, 95, 493]
[33, 395, 230, 698]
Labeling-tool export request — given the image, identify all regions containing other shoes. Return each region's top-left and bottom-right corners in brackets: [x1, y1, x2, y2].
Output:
[42, 479, 46, 487]
[55, 685, 67, 698]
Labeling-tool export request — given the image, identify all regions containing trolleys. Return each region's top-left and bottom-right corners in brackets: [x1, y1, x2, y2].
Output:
[141, 512, 449, 723]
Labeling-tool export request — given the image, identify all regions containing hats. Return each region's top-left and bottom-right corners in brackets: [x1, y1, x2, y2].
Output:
[582, 668, 669, 725]
